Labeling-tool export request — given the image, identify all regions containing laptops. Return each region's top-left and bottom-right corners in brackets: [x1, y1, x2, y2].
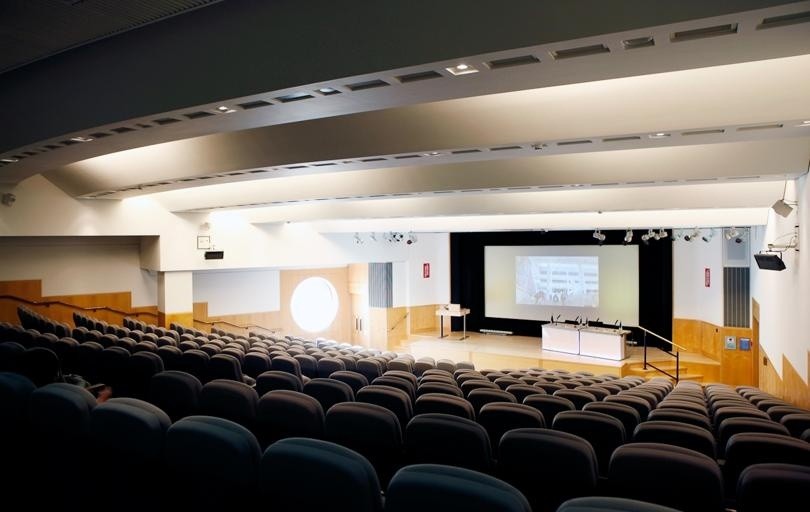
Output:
[450, 304, 462, 312]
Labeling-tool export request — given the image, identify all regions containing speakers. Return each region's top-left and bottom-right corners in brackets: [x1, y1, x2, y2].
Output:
[754, 255, 786, 271]
[206, 252, 223, 259]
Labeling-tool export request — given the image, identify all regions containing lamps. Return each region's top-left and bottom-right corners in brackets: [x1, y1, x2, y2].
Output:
[754, 243, 799, 272]
[354, 230, 419, 245]
[589, 225, 750, 244]
[773, 196, 800, 217]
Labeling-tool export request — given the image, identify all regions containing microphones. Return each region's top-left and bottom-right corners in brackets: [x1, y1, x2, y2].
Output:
[555, 314, 560, 326]
[613, 320, 618, 332]
[574, 316, 579, 328]
[595, 318, 599, 329]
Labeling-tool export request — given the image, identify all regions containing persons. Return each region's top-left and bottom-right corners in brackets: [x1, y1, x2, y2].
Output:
[49, 375, 112, 405]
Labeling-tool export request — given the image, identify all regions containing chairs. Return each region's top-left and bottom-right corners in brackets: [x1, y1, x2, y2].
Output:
[0, 304, 810, 512]
